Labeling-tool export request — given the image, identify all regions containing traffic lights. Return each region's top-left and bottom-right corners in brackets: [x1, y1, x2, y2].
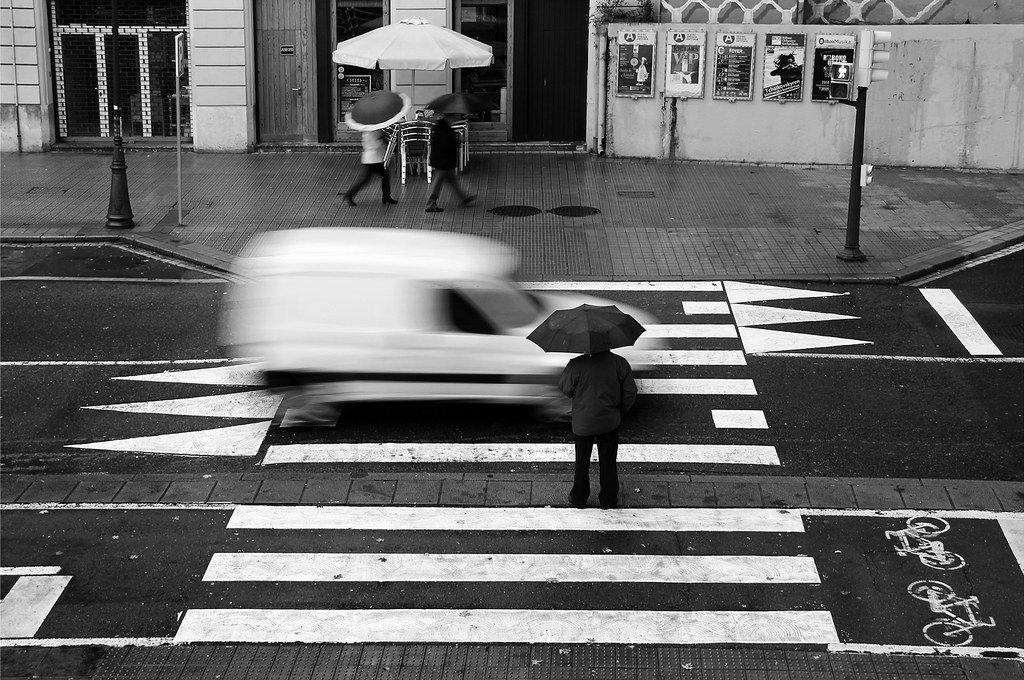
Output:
[857, 28, 891, 87]
[828, 61, 854, 100]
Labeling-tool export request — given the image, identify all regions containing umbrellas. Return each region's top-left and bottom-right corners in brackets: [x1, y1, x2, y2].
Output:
[425, 92, 489, 115]
[527, 303, 646, 353]
[344, 90, 411, 131]
[332, 16, 495, 119]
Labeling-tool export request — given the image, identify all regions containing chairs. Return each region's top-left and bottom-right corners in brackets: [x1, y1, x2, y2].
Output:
[377, 109, 469, 185]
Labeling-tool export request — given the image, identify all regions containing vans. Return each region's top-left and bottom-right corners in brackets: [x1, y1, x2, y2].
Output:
[218, 226, 670, 432]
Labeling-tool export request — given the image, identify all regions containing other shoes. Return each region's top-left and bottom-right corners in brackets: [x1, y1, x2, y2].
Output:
[425, 207, 444, 213]
[568, 495, 585, 509]
[344, 193, 357, 206]
[384, 199, 398, 204]
[458, 194, 477, 206]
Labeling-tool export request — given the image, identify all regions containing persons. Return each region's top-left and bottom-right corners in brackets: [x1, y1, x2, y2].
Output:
[558, 354, 639, 509]
[423, 113, 480, 213]
[342, 130, 399, 205]
